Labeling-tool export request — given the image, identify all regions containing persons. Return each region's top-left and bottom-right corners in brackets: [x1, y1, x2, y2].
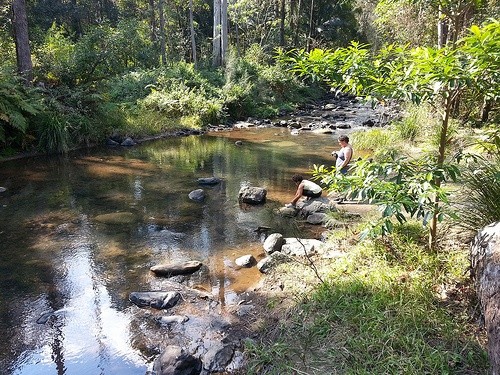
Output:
[284, 174, 322, 209]
[335, 135, 353, 176]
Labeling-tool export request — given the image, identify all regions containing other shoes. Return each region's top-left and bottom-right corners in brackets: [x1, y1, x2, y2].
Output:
[284, 203, 296, 208]
